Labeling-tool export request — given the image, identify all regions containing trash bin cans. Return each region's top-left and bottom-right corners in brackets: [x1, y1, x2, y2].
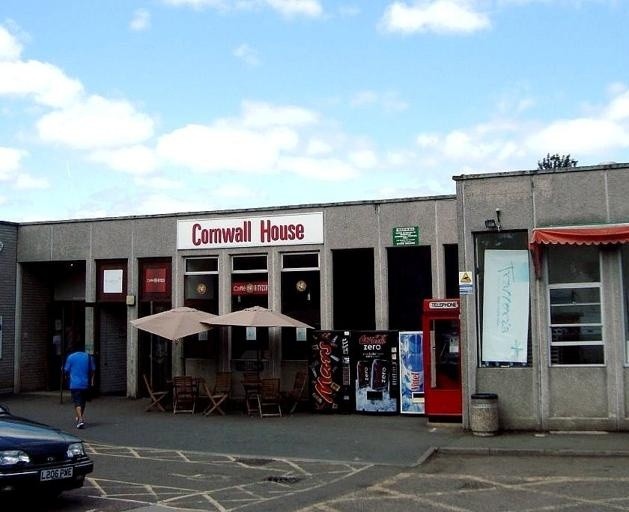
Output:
[470, 393, 499, 437]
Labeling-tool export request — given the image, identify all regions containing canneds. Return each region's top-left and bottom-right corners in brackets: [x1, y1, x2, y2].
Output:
[371, 359, 389, 390]
[357, 361, 370, 388]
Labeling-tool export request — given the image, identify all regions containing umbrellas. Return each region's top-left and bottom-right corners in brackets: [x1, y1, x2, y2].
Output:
[127, 305, 218, 339]
[202, 304, 317, 379]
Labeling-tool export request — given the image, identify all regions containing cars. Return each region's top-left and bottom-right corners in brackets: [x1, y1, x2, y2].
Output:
[0, 400, 95, 508]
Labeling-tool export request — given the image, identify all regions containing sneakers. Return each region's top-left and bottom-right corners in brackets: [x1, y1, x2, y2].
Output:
[75, 416, 86, 428]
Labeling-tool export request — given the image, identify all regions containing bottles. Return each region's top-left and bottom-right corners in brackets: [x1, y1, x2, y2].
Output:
[401, 334, 423, 400]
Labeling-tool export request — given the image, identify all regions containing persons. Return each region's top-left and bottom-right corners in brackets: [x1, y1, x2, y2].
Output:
[63, 340, 97, 429]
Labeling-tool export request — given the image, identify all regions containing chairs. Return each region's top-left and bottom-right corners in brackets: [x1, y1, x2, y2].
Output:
[142, 370, 308, 420]
